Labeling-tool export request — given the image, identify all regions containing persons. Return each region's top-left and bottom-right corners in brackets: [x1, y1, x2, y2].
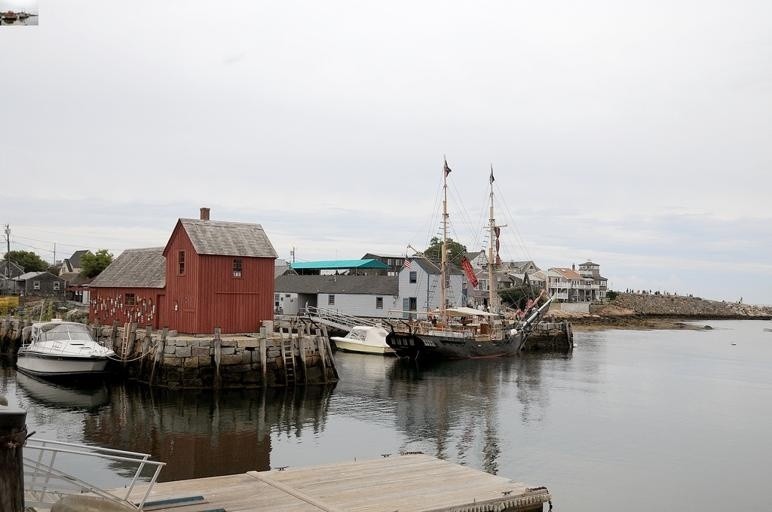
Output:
[304, 300, 310, 317]
[428, 307, 441, 327]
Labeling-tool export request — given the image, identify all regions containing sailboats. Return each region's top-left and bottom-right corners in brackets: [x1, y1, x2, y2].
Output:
[385, 153, 533, 361]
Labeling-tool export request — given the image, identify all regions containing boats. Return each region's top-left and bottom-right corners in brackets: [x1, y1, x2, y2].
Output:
[330, 326, 395, 354]
[15, 371, 108, 408]
[15, 318, 114, 375]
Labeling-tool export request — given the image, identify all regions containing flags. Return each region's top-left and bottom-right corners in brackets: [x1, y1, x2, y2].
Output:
[403, 255, 412, 269]
